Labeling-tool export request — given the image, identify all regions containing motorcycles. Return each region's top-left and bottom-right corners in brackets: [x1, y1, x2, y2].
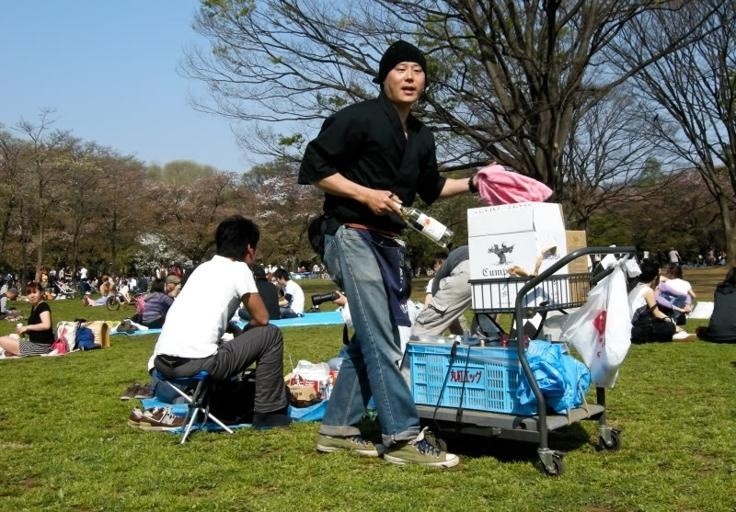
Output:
[106, 275, 146, 311]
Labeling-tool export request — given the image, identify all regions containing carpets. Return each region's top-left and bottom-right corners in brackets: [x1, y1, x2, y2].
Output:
[108, 309, 347, 336]
[0, 349, 80, 360]
[139, 394, 377, 433]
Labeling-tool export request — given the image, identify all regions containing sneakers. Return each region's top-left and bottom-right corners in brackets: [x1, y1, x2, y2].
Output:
[127, 407, 192, 432]
[672, 331, 697, 342]
[316, 434, 460, 470]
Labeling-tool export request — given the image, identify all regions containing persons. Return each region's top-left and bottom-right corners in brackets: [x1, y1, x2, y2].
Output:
[300, 39, 497, 468]
[155, 215, 292, 431]
[238, 264, 304, 321]
[399, 243, 498, 391]
[0, 263, 196, 357]
[593, 244, 736, 343]
[331, 287, 419, 366]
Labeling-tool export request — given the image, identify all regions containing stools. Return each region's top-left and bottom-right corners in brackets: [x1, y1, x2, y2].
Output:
[152, 369, 235, 445]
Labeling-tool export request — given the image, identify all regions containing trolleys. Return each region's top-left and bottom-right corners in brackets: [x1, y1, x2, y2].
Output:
[412, 241, 638, 476]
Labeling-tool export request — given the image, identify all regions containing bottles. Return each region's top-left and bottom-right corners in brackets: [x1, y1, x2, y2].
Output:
[393, 201, 454, 248]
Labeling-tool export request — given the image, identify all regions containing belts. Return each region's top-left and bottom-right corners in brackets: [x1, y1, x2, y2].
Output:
[157, 356, 190, 368]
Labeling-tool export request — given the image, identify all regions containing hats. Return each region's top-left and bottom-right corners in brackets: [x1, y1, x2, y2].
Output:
[372, 40, 427, 83]
